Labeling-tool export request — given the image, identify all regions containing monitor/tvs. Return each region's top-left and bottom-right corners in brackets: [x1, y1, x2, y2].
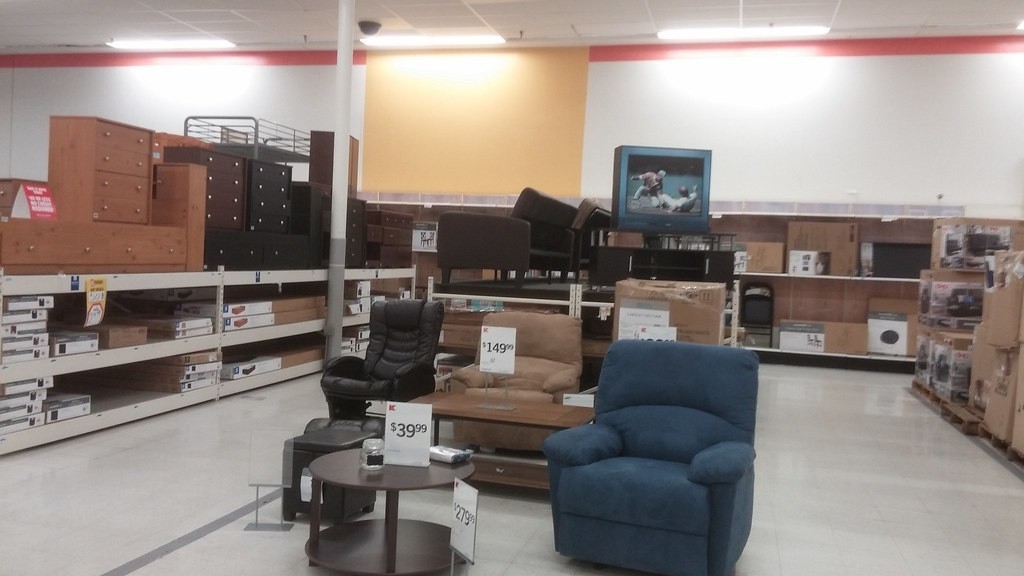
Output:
[611, 145, 712, 236]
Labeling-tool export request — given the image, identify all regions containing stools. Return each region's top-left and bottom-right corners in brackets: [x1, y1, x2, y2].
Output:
[282, 429, 378, 527]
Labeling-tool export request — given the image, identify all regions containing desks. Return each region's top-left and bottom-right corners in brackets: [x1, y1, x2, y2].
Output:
[406, 391, 596, 490]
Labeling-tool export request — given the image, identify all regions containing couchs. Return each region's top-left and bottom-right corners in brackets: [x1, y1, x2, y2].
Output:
[321, 186, 759, 576]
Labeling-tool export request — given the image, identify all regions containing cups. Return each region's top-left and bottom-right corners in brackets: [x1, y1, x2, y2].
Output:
[360, 439, 386, 470]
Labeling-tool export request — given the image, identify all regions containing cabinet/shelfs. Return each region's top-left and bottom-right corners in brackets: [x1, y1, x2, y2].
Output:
[0, 267, 417, 457]
[0, 115, 425, 275]
[725, 214, 933, 370]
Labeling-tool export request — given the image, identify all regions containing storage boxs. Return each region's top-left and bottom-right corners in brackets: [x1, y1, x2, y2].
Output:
[611, 276, 726, 345]
[0, 277, 412, 436]
[734, 216, 1024, 461]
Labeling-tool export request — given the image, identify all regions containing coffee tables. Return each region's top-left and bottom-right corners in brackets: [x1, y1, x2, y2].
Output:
[305, 445, 476, 576]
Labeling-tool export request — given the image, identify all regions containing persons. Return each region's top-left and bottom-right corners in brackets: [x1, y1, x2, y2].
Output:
[631, 170, 698, 213]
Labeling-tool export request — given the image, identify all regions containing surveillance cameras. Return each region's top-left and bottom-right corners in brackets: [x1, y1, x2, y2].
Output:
[358, 21, 382, 36]
